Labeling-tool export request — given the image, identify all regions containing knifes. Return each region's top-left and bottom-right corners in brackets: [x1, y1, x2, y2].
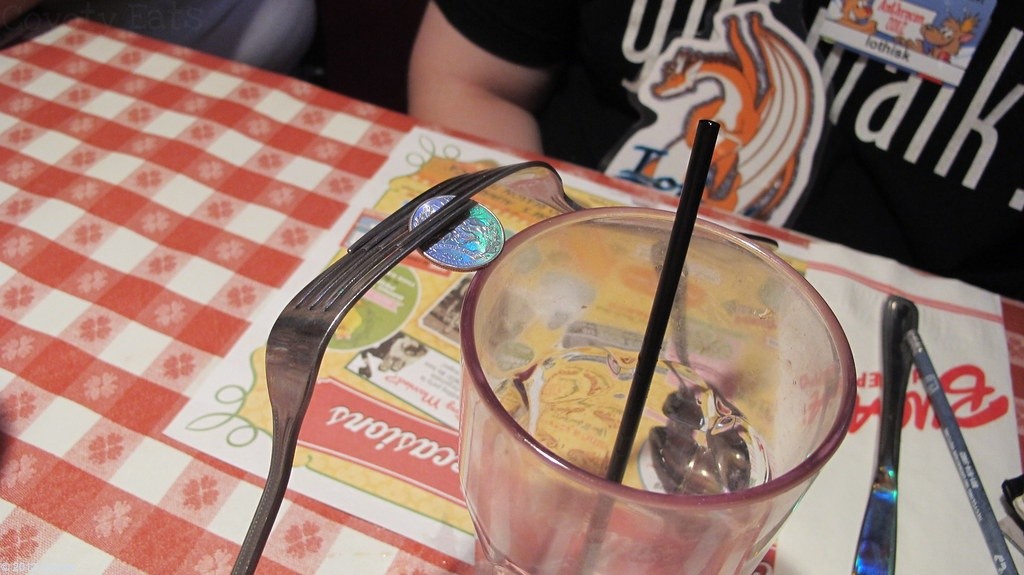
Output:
[851, 294, 920, 575]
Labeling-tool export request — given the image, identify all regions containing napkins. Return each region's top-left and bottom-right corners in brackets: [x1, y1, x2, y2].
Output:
[770, 240, 1024, 575]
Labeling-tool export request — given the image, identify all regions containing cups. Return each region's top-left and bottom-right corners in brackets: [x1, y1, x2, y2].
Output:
[459, 206, 857, 575]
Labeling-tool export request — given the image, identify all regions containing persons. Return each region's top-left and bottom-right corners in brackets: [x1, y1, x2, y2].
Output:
[407, 0, 1024, 301]
[361, 331, 426, 379]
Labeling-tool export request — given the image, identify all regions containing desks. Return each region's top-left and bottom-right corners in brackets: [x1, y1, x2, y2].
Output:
[1, 18, 1024, 575]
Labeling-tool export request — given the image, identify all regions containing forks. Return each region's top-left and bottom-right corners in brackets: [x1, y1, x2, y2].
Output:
[346, 160, 779, 254]
[230, 200, 478, 575]
